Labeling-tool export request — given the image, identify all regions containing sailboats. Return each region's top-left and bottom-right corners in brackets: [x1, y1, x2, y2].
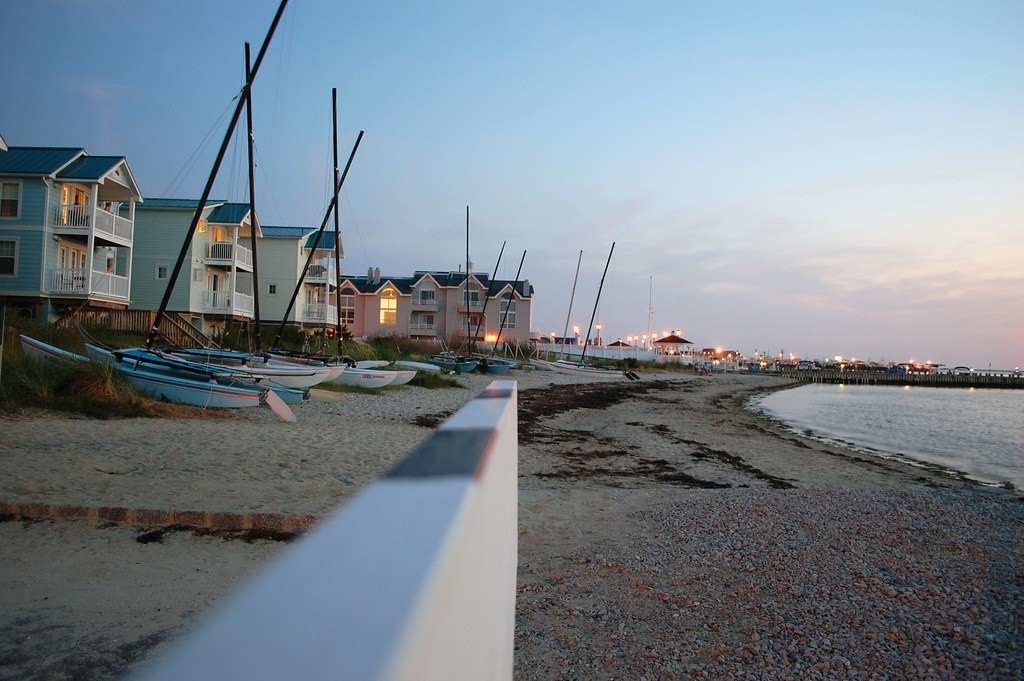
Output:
[19, 0, 641, 425]
